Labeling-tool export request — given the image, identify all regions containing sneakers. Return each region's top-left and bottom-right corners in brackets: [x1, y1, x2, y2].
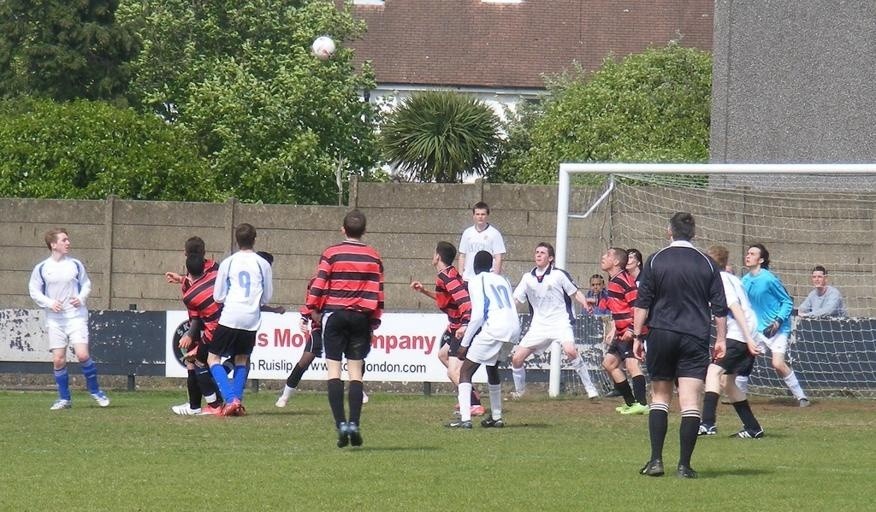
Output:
[91, 390, 110, 407]
[337, 421, 349, 447]
[49, 399, 72, 410]
[678, 464, 697, 478]
[589, 389, 810, 439]
[349, 422, 363, 446]
[171, 398, 287, 416]
[639, 457, 665, 476]
[445, 392, 525, 428]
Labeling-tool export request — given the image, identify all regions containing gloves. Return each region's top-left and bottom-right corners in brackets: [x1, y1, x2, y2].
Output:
[763, 316, 783, 338]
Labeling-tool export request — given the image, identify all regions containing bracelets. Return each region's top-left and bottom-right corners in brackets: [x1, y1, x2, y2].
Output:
[633, 333, 645, 339]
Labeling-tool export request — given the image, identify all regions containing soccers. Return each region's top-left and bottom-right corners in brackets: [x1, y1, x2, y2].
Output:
[313, 36, 335, 58]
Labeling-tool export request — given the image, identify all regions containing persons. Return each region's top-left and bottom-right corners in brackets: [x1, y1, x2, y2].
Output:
[444, 251, 522, 428]
[512, 241, 602, 401]
[697, 246, 766, 439]
[408, 239, 487, 418]
[275, 276, 371, 409]
[625, 249, 644, 290]
[178, 252, 286, 416]
[164, 236, 222, 416]
[581, 274, 609, 316]
[308, 208, 384, 448]
[797, 266, 848, 319]
[27, 227, 111, 412]
[205, 224, 275, 415]
[457, 200, 506, 294]
[721, 244, 811, 409]
[583, 247, 650, 415]
[631, 210, 728, 478]
